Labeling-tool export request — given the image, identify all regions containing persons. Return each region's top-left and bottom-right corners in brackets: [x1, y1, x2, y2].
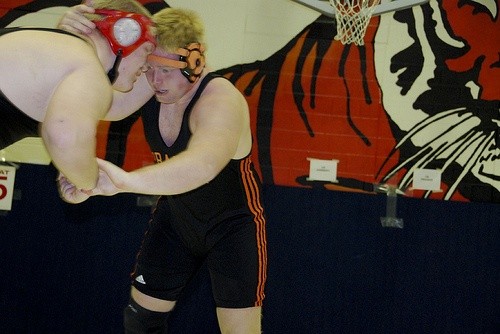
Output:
[0, 0, 158, 192]
[55, 5, 267, 334]
